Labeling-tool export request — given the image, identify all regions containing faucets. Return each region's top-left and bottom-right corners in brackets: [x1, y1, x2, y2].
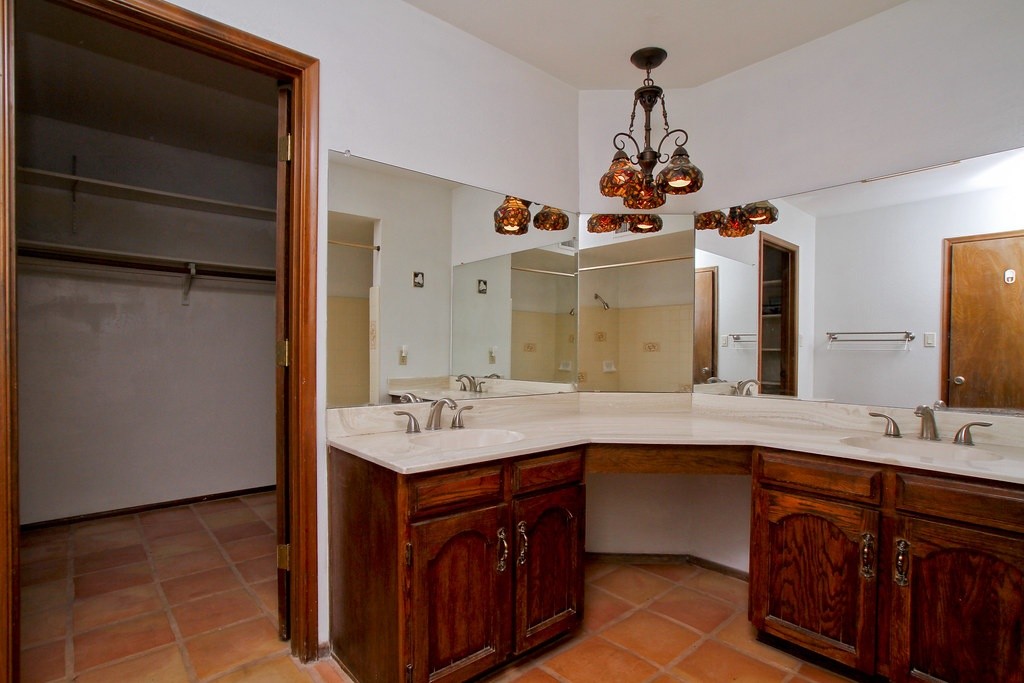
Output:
[707, 377, 726, 384]
[738, 379, 760, 396]
[400, 393, 418, 403]
[914, 405, 941, 441]
[489, 373, 500, 379]
[456, 374, 477, 391]
[425, 398, 458, 430]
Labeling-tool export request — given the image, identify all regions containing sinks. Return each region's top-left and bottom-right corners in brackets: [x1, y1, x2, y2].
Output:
[839, 436, 1006, 463]
[411, 429, 524, 450]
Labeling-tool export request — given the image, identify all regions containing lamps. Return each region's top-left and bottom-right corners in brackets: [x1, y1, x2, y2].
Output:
[599, 47, 703, 210]
[695, 200, 779, 237]
[586, 214, 662, 233]
[494, 194, 570, 236]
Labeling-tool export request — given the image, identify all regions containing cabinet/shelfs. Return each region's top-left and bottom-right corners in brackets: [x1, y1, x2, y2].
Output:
[328, 445, 587, 683]
[747, 447, 1024, 683]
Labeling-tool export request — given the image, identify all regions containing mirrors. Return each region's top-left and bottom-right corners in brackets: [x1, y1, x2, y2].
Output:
[692, 146, 1024, 418]
[578, 213, 695, 394]
[511, 239, 579, 385]
[327, 148, 578, 410]
